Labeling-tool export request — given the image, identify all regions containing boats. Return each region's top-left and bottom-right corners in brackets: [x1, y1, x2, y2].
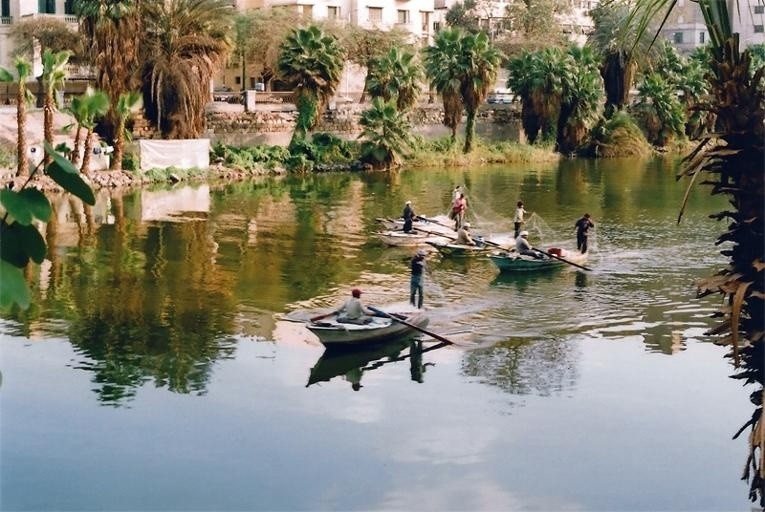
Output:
[486, 248, 588, 272]
[375, 216, 518, 258]
[306, 310, 429, 342]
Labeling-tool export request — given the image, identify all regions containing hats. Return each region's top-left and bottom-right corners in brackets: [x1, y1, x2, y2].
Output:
[352, 289, 363, 294]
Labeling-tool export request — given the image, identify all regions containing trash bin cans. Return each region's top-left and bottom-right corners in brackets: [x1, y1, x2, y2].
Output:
[241, 90, 256, 113]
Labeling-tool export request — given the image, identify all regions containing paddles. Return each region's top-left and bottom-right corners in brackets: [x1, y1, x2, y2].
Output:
[368, 306, 453, 345]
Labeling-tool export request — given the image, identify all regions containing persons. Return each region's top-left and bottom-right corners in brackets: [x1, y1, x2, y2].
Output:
[452, 193, 466, 232]
[514, 201, 536, 240]
[409, 249, 433, 310]
[572, 270, 590, 302]
[515, 230, 545, 259]
[457, 222, 476, 246]
[570, 213, 594, 255]
[451, 185, 462, 207]
[402, 200, 418, 235]
[409, 340, 435, 384]
[336, 288, 374, 326]
[344, 361, 369, 392]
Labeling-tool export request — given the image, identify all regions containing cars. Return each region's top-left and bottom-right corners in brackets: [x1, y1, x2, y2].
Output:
[488, 88, 518, 104]
[213, 88, 264, 105]
